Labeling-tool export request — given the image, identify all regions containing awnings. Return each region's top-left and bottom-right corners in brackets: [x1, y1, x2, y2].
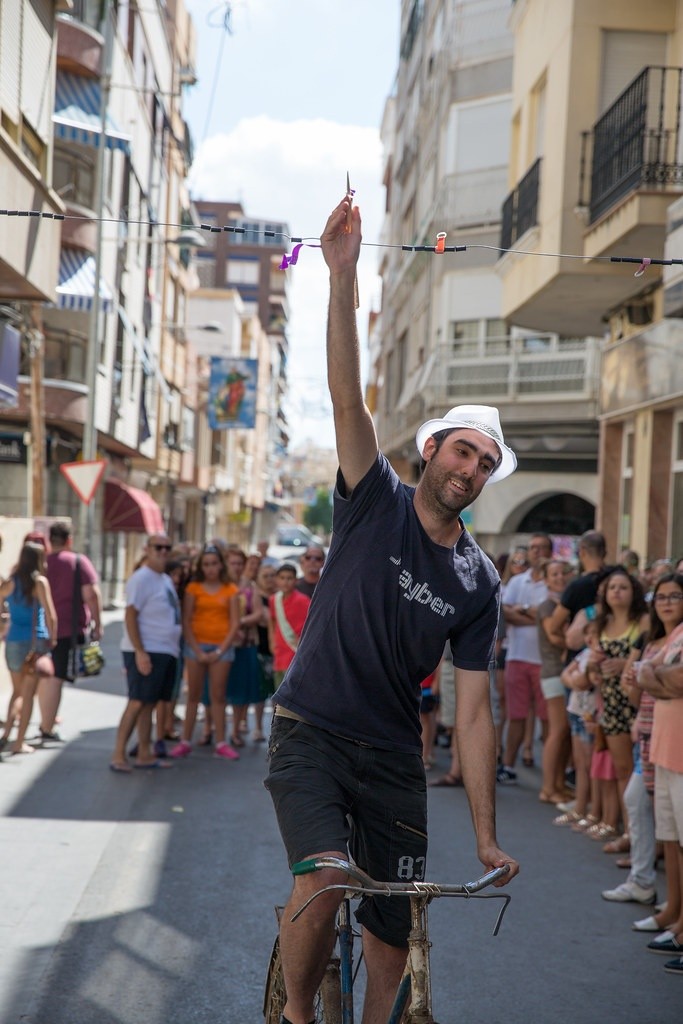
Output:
[39, 245, 113, 314]
[51, 66, 133, 153]
[100, 478, 164, 536]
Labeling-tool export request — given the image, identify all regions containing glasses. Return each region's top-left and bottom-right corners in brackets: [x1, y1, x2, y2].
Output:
[203, 546, 216, 552]
[304, 554, 324, 562]
[147, 544, 173, 552]
[656, 594, 680, 604]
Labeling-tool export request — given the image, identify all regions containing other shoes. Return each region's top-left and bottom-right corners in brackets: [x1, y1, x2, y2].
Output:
[7, 720, 266, 776]
[420, 735, 642, 870]
[648, 939, 683, 954]
[663, 957, 681, 974]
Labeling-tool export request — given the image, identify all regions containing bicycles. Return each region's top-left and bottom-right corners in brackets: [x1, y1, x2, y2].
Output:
[263, 855, 513, 1024]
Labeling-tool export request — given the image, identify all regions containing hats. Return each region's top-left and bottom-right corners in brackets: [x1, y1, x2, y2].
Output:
[415, 404, 517, 487]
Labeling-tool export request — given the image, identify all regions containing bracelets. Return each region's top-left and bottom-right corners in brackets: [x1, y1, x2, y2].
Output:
[215, 649, 222, 656]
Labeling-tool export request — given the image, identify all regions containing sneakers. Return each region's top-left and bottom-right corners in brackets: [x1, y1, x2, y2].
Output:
[602, 880, 658, 907]
[654, 901, 666, 911]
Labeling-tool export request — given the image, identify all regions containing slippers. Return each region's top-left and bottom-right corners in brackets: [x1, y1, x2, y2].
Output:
[656, 929, 676, 944]
[632, 916, 666, 930]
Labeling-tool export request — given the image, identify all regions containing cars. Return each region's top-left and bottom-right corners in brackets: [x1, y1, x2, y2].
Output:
[261, 522, 328, 579]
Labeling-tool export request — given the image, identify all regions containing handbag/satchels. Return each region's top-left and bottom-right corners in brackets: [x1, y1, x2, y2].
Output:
[233, 636, 263, 704]
[65, 642, 104, 683]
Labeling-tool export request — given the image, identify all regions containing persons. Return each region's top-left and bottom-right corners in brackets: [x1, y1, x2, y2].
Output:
[500, 533, 553, 783]
[128, 538, 276, 760]
[0, 531, 58, 764]
[295, 544, 325, 597]
[549, 530, 683, 974]
[414, 553, 509, 786]
[535, 560, 572, 805]
[109, 534, 182, 773]
[167, 543, 242, 760]
[268, 564, 312, 690]
[38, 521, 103, 746]
[263, 186, 519, 1024]
